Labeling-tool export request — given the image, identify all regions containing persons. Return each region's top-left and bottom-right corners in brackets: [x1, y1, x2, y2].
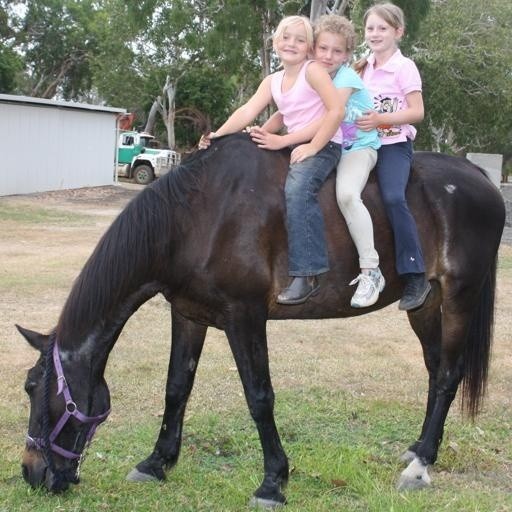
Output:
[245, 10, 390, 310]
[196, 14, 346, 306]
[350, 1, 433, 311]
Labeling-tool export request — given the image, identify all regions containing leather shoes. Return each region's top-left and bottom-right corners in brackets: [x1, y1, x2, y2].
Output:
[276, 276, 321, 305]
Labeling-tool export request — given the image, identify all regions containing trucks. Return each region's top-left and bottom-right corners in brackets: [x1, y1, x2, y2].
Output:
[115, 125, 184, 185]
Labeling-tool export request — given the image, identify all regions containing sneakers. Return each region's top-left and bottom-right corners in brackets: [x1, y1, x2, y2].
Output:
[348, 268, 386, 309]
[399, 273, 432, 310]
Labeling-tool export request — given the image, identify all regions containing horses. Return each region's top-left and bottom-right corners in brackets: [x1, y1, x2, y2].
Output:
[14, 130, 506, 506]
